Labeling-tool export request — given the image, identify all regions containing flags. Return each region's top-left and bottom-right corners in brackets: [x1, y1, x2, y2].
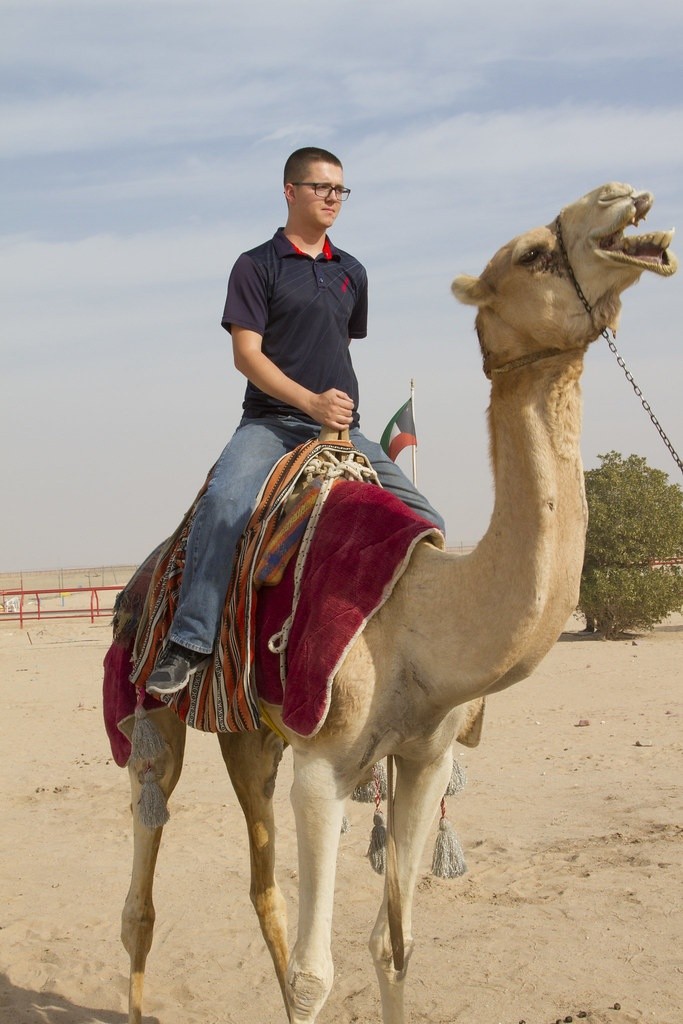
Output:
[380, 396, 418, 462]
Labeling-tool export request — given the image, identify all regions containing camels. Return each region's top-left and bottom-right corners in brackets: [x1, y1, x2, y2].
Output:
[105, 183, 679, 1024]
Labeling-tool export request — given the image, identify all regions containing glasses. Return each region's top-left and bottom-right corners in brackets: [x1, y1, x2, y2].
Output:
[283, 181, 352, 202]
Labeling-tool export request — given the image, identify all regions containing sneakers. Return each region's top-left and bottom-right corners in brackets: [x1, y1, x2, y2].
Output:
[144, 639, 212, 695]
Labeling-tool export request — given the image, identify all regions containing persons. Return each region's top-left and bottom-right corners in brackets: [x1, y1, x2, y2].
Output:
[146, 148, 445, 694]
